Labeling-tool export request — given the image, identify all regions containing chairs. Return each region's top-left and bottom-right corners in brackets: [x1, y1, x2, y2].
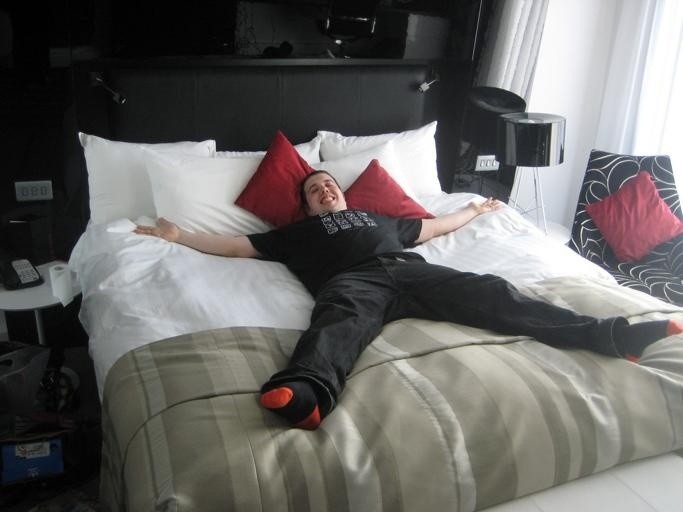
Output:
[570, 149, 683, 305]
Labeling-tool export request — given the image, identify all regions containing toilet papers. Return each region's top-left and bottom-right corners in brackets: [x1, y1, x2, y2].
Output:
[49, 264, 75, 309]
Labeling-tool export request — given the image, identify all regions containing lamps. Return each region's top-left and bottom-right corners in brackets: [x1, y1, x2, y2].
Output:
[494, 114, 566, 236]
[91, 73, 127, 106]
[418, 73, 441, 93]
[451, 84, 525, 194]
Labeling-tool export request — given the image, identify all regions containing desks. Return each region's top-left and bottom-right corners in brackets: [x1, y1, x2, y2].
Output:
[0, 260, 82, 347]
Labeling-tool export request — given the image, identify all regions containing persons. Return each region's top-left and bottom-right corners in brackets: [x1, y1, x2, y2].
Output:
[131, 169, 683, 431]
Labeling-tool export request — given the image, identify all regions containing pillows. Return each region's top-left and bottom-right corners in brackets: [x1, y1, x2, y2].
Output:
[77, 132, 216, 230]
[308, 140, 416, 211]
[343, 159, 437, 219]
[317, 121, 442, 211]
[234, 130, 316, 227]
[217, 136, 323, 163]
[144, 157, 267, 234]
[585, 172, 682, 264]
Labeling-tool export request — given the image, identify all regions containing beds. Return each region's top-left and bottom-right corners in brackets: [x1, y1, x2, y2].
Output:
[66, 60, 683, 512]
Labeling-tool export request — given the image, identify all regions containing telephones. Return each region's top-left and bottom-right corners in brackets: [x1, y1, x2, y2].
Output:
[0, 253, 44, 290]
